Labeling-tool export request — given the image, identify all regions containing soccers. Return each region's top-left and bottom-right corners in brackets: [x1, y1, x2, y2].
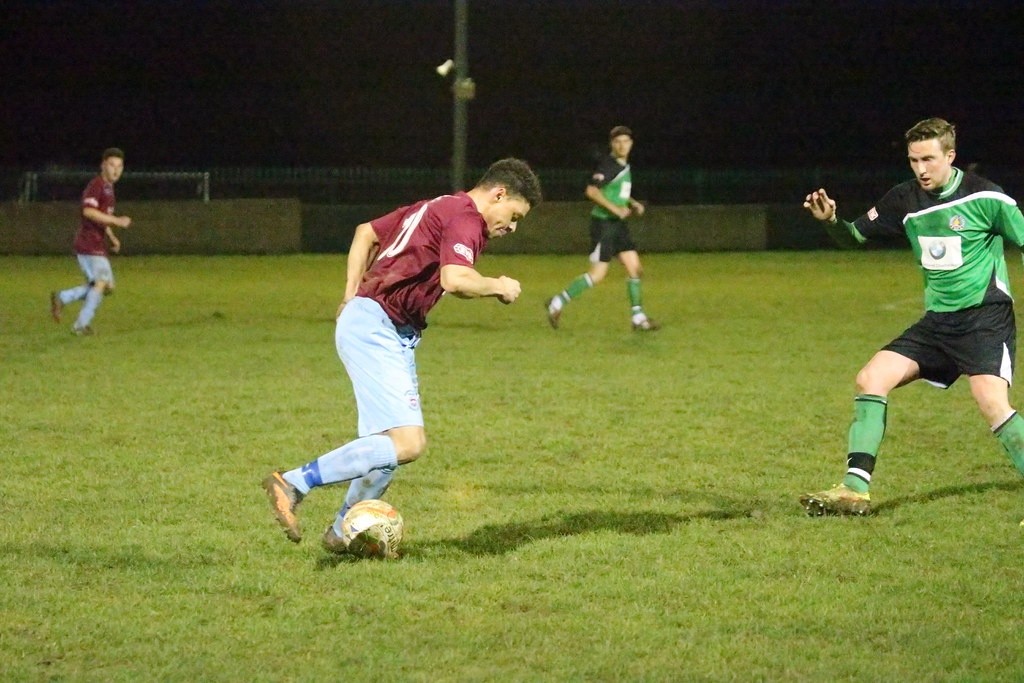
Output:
[341, 499, 405, 562]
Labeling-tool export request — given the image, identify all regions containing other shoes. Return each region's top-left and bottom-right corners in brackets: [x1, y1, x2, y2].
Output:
[51, 291, 63, 323]
[632, 317, 660, 330]
[545, 297, 560, 329]
[71, 327, 92, 336]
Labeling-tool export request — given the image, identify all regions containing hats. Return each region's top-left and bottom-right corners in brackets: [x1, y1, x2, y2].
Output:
[609, 126, 633, 139]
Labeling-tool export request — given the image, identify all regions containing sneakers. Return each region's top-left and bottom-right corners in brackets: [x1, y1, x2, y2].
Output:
[264, 468, 304, 543]
[323, 526, 410, 561]
[798, 483, 873, 516]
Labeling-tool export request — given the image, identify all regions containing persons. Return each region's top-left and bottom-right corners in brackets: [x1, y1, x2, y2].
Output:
[544, 125, 661, 331]
[798, 117, 1023, 516]
[50, 148, 131, 336]
[260, 159, 544, 555]
